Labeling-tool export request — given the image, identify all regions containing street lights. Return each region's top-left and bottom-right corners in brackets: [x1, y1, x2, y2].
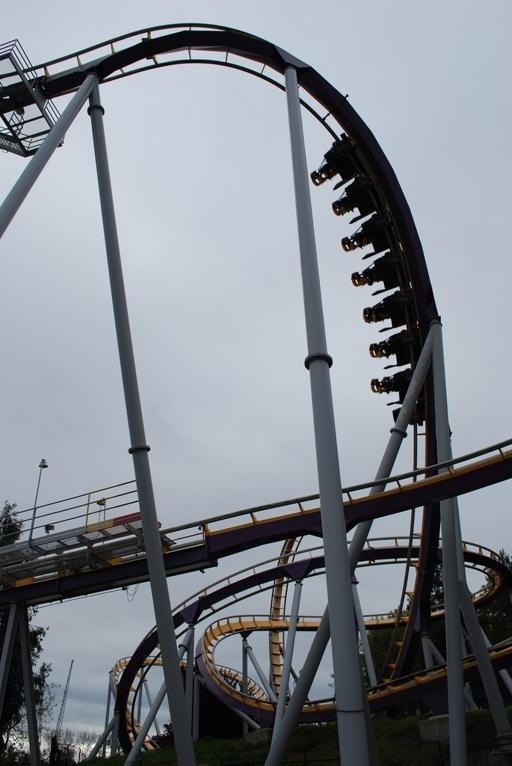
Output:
[27, 458, 48, 540]
[96, 497, 106, 545]
